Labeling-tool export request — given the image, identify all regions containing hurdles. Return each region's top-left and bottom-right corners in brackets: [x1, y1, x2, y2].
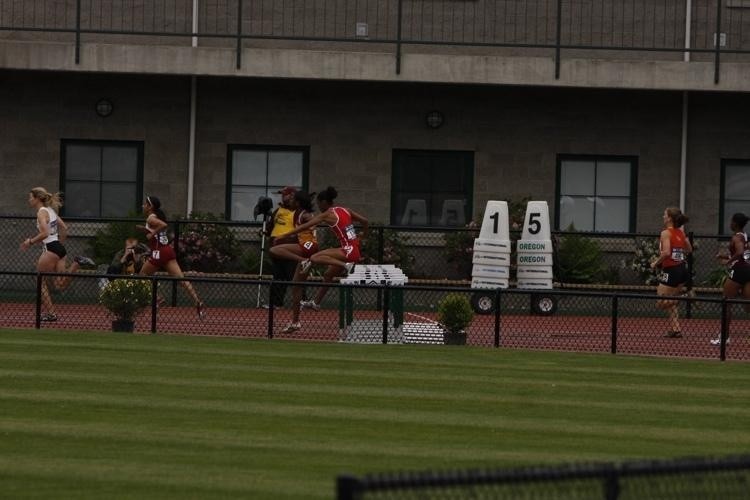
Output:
[339, 265, 408, 342]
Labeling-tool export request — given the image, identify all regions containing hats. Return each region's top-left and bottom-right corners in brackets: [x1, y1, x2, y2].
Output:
[278, 185, 298, 195]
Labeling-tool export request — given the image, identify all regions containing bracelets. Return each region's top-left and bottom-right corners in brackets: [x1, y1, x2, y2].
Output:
[29, 237, 33, 245]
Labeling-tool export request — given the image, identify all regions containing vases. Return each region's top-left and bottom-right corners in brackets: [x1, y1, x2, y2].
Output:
[109, 316, 135, 333]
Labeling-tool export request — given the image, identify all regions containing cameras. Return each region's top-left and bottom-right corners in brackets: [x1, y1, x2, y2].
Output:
[254, 197, 275, 216]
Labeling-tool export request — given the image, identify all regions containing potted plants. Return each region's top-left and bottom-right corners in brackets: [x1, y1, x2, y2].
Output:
[437, 290, 477, 345]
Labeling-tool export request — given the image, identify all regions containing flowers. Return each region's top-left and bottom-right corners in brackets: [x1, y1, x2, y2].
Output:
[100, 275, 157, 321]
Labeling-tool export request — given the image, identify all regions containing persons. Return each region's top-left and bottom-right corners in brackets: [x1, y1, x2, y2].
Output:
[269, 191, 318, 333]
[273, 186, 368, 311]
[107, 237, 143, 281]
[18, 187, 95, 322]
[649, 207, 693, 337]
[711, 213, 750, 346]
[259, 185, 296, 309]
[136, 196, 208, 323]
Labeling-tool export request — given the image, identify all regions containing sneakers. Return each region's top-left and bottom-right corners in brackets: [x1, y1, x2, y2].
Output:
[345, 261, 356, 274]
[298, 257, 312, 274]
[281, 321, 303, 333]
[40, 311, 57, 321]
[262, 303, 282, 310]
[663, 330, 683, 338]
[300, 299, 322, 311]
[73, 254, 95, 266]
[710, 333, 730, 346]
[196, 300, 207, 320]
